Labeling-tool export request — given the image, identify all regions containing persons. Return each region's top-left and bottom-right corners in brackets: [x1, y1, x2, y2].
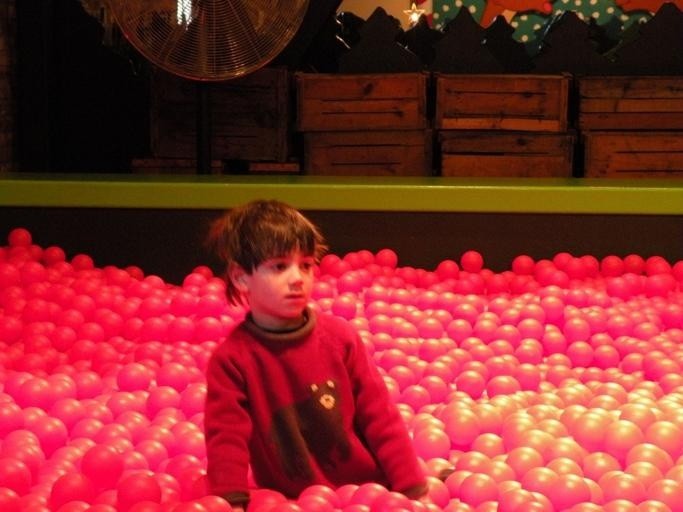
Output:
[201, 195, 431, 512]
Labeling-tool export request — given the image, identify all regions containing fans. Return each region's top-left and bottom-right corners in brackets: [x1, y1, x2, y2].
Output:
[106, 1, 311, 176]
